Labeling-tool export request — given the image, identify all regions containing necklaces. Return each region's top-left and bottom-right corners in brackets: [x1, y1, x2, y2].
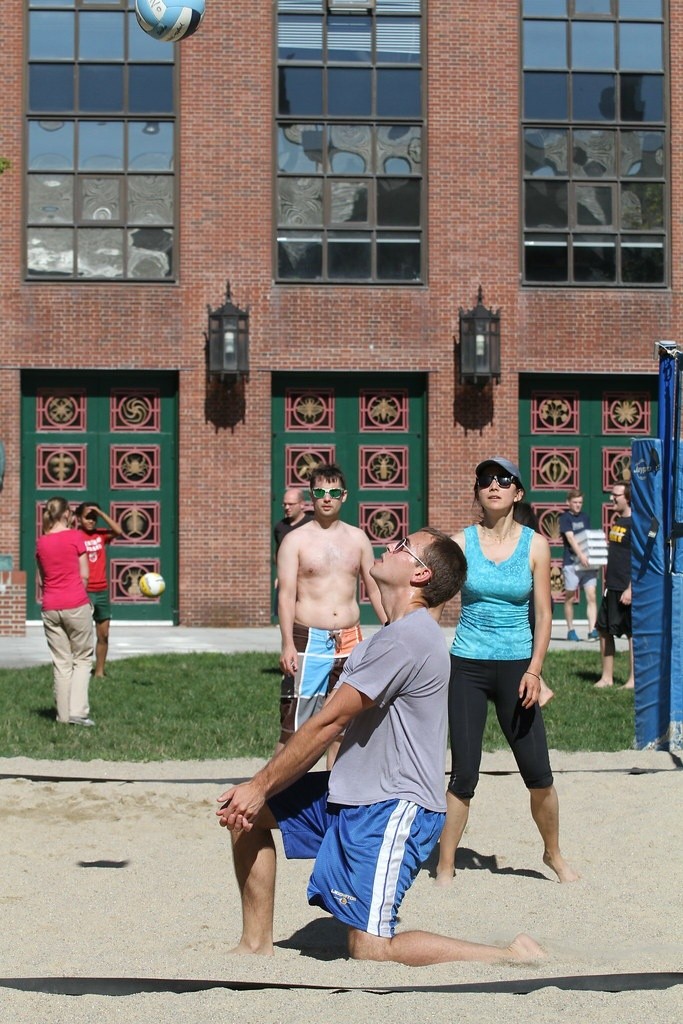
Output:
[481, 521, 516, 542]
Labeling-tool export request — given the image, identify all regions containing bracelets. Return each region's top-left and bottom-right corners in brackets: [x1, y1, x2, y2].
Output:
[526, 671, 540, 680]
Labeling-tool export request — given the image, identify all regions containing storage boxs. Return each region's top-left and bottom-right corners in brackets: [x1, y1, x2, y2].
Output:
[574, 532, 608, 572]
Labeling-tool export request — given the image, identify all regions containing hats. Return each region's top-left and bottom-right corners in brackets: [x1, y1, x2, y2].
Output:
[475, 456, 522, 486]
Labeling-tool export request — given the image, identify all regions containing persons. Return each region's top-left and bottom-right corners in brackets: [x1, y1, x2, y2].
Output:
[76, 502, 124, 678]
[426, 457, 577, 883]
[559, 487, 599, 642]
[590, 480, 635, 689]
[217, 517, 550, 968]
[276, 465, 390, 772]
[36, 497, 99, 726]
[274, 489, 317, 615]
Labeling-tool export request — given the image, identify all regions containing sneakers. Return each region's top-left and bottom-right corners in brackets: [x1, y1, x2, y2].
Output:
[69, 714, 96, 727]
[588, 630, 601, 641]
[568, 630, 583, 642]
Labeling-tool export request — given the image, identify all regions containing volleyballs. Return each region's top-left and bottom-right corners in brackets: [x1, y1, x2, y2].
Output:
[134, 0, 207, 43]
[138, 572, 166, 598]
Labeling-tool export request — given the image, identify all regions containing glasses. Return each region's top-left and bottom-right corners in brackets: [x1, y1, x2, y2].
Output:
[475, 473, 513, 488]
[394, 538, 430, 572]
[281, 503, 296, 507]
[311, 487, 344, 499]
[610, 493, 625, 499]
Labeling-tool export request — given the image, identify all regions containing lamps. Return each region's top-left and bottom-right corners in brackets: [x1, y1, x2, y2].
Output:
[206, 280, 251, 387]
[455, 284, 502, 392]
